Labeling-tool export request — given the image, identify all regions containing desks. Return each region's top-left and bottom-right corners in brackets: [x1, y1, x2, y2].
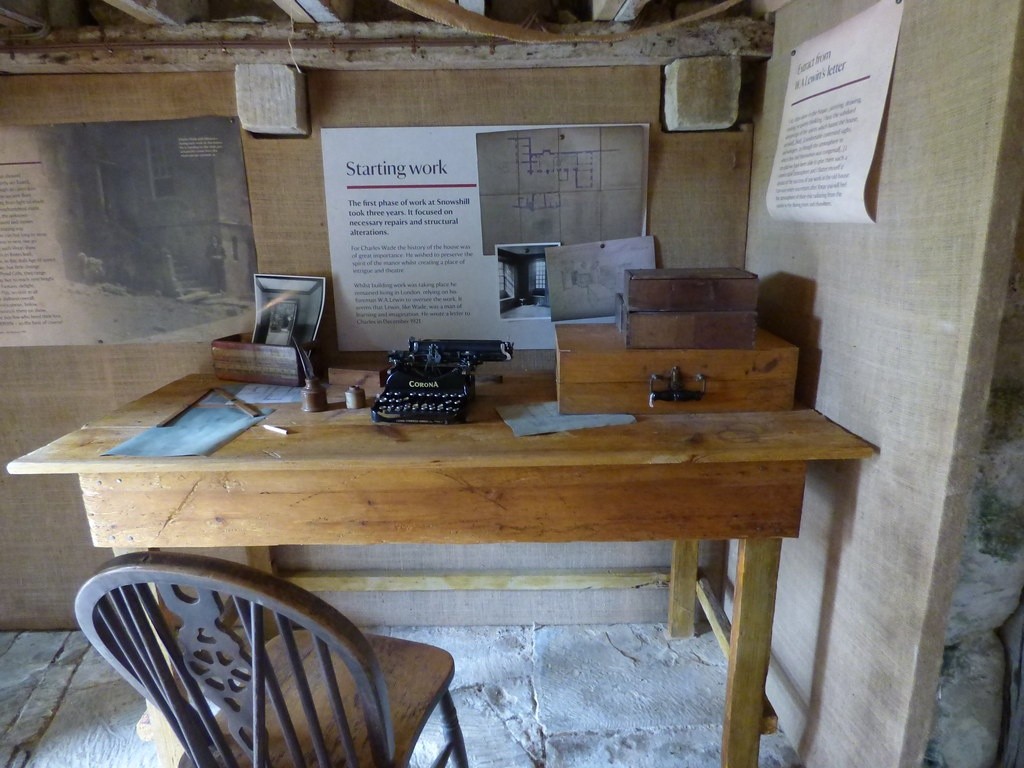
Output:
[6, 369, 875, 768]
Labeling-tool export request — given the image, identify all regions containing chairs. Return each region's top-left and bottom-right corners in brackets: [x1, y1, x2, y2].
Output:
[74, 551, 470, 767]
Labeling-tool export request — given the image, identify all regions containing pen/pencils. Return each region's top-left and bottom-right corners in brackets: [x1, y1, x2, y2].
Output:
[261, 423, 287, 435]
